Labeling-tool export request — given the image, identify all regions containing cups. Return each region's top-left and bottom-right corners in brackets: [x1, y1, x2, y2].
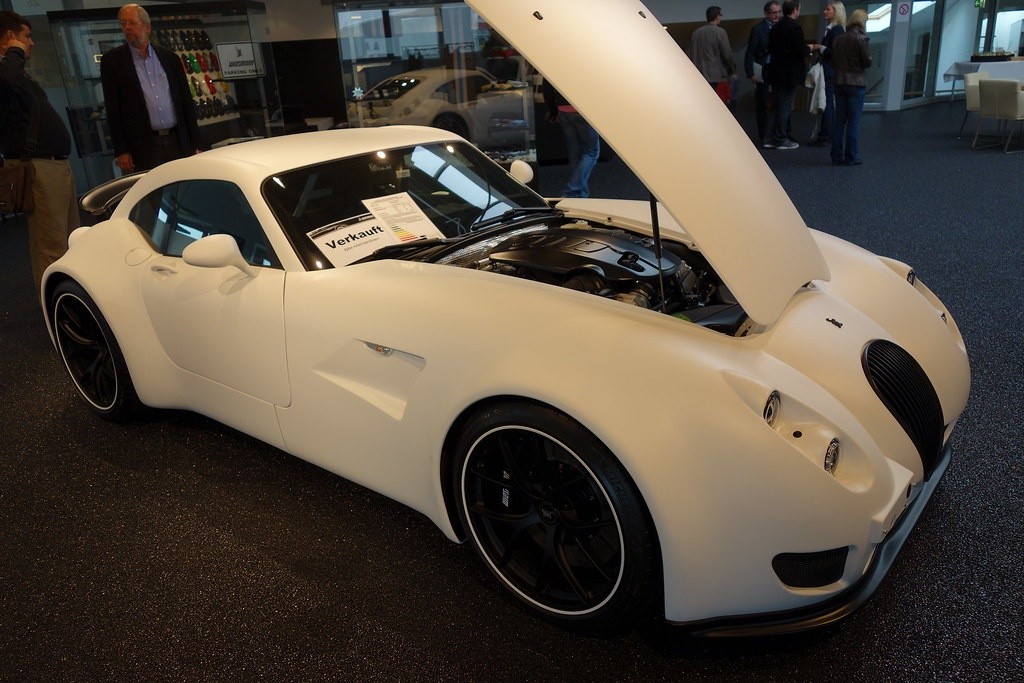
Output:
[996, 47, 1003, 56]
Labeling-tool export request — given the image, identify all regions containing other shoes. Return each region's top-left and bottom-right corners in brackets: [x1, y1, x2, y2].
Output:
[850, 158, 862, 165]
[831, 160, 840, 165]
[808, 139, 829, 146]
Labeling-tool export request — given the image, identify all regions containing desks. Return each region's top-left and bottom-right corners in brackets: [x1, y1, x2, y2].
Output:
[943, 60, 1024, 100]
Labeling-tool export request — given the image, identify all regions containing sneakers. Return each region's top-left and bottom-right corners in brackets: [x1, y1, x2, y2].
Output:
[776, 138, 799, 150]
[762, 139, 779, 148]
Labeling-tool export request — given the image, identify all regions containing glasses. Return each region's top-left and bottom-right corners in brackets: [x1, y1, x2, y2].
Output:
[718, 13, 723, 16]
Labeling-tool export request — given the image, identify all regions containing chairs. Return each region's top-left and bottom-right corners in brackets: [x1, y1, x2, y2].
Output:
[971, 78, 1024, 153]
[957, 72, 1008, 145]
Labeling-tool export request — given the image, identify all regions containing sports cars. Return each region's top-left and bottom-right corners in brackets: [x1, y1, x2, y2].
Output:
[40, 0, 975, 649]
[345, 66, 528, 154]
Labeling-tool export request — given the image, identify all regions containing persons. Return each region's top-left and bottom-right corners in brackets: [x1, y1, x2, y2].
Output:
[555, 83, 601, 202]
[99, 3, 195, 195]
[2, 12, 81, 311]
[688, 1, 872, 164]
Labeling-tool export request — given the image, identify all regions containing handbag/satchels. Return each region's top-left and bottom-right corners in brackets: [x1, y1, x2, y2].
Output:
[0, 159, 35, 216]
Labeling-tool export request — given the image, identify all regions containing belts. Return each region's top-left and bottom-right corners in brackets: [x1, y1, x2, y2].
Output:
[151, 126, 178, 136]
[4, 155, 69, 160]
